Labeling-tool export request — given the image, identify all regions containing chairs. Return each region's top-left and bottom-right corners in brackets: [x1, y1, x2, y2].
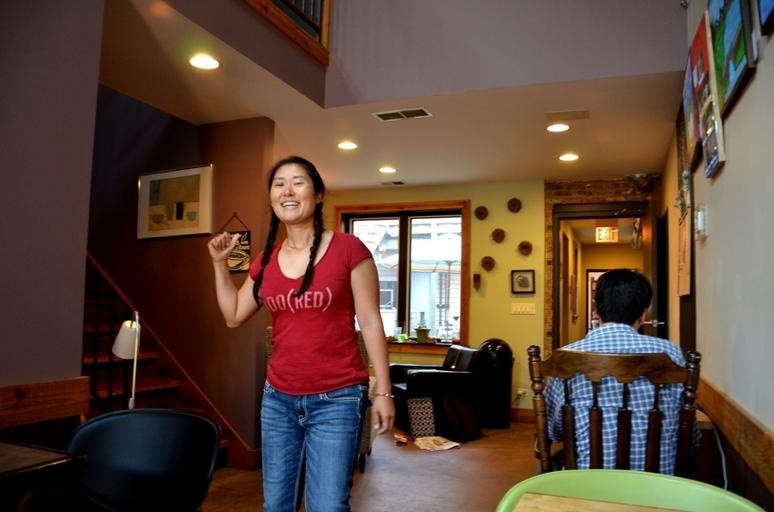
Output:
[265, 326, 376, 473]
[527, 345, 701, 477]
[60, 408, 222, 512]
[496, 469, 767, 512]
[389, 344, 483, 434]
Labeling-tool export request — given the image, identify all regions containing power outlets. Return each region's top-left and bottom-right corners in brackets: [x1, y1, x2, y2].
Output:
[517, 388, 529, 397]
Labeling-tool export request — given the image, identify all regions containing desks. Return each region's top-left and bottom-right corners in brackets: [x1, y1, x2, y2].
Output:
[512, 492, 690, 512]
[0, 440, 89, 512]
[695, 409, 713, 430]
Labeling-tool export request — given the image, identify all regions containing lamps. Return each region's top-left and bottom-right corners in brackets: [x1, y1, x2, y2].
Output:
[595, 227, 619, 244]
[112, 311, 141, 408]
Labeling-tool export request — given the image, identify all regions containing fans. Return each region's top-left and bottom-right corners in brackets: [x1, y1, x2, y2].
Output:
[480, 339, 511, 359]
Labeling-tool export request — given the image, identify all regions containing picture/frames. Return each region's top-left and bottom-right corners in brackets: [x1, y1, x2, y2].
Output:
[511, 270, 535, 294]
[137, 164, 215, 240]
[683, 0, 774, 178]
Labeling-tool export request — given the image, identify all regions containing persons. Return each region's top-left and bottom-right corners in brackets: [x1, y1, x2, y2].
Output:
[207, 156, 398, 512]
[542, 266, 687, 478]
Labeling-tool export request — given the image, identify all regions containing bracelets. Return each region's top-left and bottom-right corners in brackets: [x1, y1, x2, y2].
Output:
[371, 392, 395, 399]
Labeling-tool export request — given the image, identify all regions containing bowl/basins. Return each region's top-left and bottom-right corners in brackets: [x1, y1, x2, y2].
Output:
[226, 251, 245, 268]
